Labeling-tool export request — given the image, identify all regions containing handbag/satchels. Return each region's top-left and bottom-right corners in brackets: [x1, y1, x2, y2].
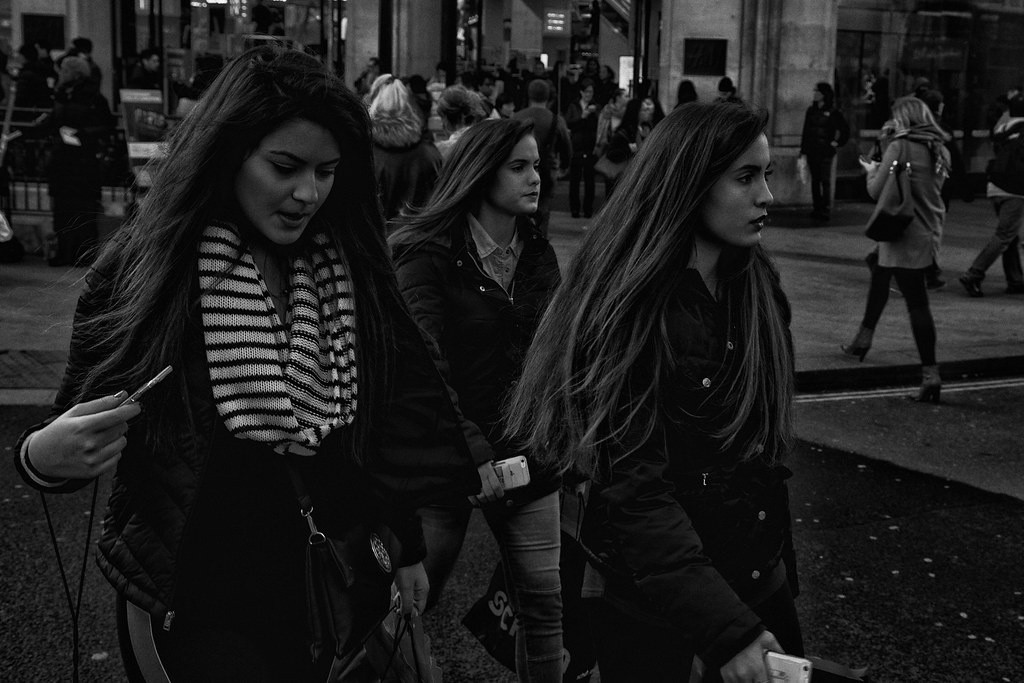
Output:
[93, 128, 136, 188]
[863, 138, 915, 242]
[594, 142, 608, 158]
[327, 581, 442, 683]
[538, 114, 558, 182]
[460, 485, 617, 683]
[594, 128, 637, 180]
[305, 521, 404, 664]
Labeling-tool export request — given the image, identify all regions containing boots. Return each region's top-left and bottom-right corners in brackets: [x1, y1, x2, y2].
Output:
[910, 365, 942, 403]
[840, 322, 874, 362]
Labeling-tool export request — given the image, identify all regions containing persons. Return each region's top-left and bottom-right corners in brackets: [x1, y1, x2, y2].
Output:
[13, 45, 428, 683]
[381, 114, 567, 683]
[501, 100, 812, 683]
[798, 75, 1024, 404]
[0, 37, 743, 270]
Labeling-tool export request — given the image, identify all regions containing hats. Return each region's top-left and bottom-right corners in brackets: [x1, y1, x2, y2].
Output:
[61, 56, 91, 78]
[363, 73, 409, 120]
[18, 42, 40, 61]
[718, 77, 733, 92]
[914, 77, 929, 90]
[72, 37, 93, 54]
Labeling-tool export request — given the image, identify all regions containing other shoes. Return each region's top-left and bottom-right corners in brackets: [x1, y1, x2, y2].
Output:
[866, 252, 878, 271]
[47, 244, 99, 266]
[927, 278, 946, 289]
[1003, 280, 1024, 294]
[959, 266, 985, 295]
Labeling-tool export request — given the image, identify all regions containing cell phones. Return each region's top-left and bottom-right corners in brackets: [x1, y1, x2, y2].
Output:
[764, 650, 813, 683]
[115, 365, 175, 409]
[859, 154, 872, 166]
[491, 454, 531, 491]
